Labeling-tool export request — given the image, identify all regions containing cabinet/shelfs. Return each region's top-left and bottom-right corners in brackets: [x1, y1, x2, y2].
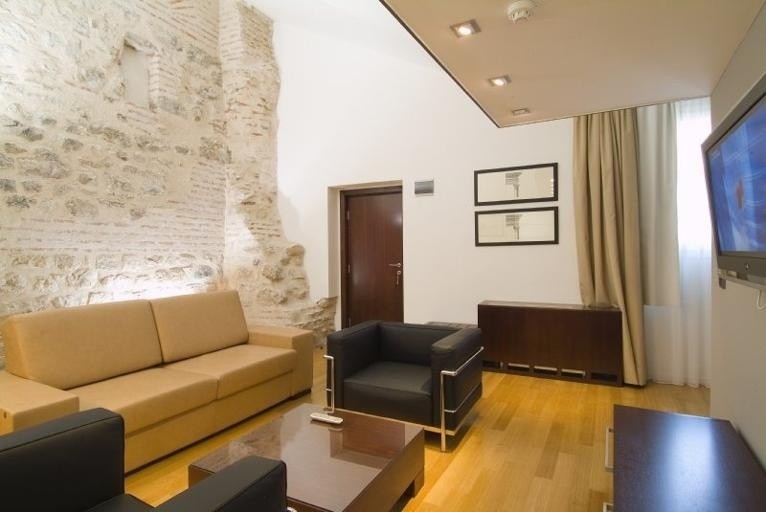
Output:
[603, 404, 766, 511]
[476, 299, 623, 386]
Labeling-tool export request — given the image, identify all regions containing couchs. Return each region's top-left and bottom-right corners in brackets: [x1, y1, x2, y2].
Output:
[0, 290, 316, 473]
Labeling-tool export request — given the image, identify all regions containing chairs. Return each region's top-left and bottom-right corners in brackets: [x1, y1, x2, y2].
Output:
[323, 320, 484, 451]
[0, 406, 289, 512]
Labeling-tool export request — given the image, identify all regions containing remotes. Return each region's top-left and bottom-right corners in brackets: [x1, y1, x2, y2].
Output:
[311, 412, 343, 424]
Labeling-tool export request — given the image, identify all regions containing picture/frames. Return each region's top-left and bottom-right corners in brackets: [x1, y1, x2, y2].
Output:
[474, 162, 558, 206]
[474, 206, 559, 246]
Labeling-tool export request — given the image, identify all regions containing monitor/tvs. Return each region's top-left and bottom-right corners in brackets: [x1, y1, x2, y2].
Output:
[700, 71, 766, 292]
